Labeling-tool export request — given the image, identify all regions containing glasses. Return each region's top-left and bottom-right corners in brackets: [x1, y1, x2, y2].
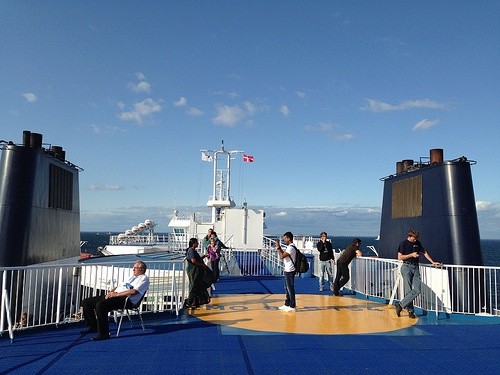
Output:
[134, 266, 139, 269]
[321, 236, 326, 237]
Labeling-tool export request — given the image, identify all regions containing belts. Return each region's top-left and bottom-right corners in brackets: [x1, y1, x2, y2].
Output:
[403, 262, 418, 266]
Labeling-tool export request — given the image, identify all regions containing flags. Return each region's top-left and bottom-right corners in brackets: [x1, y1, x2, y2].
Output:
[243, 154, 254, 162]
[201, 152, 213, 162]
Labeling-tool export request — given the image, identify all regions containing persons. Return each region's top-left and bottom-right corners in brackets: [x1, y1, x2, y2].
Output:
[5, 312, 27, 329]
[316, 232, 335, 292]
[395, 228, 440, 318]
[333, 238, 362, 297]
[202, 228, 229, 283]
[275, 232, 297, 311]
[182, 238, 210, 307]
[79, 261, 150, 341]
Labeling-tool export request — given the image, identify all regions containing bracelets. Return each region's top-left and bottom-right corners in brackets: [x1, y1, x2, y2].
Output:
[276, 248, 281, 250]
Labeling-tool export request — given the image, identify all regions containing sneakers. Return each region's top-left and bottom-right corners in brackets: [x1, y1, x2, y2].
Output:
[278, 305, 291, 310]
[284, 307, 296, 312]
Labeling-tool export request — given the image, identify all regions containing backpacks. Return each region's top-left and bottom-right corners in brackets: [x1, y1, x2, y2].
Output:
[288, 245, 309, 277]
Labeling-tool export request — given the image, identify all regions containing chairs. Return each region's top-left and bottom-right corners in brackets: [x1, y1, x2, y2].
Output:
[114, 289, 147, 337]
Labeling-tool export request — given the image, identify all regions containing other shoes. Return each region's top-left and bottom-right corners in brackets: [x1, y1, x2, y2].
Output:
[395, 301, 402, 317]
[186, 314, 196, 318]
[80, 329, 97, 334]
[333, 292, 343, 296]
[330, 283, 335, 292]
[93, 334, 110, 340]
[320, 288, 324, 291]
[408, 310, 415, 318]
